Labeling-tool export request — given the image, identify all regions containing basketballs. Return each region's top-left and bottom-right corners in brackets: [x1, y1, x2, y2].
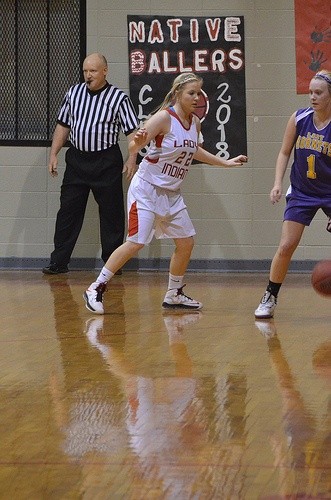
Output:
[311, 260, 331, 300]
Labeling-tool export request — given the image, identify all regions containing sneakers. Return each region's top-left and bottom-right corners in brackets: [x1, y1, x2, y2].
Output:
[42, 263, 69, 274]
[162, 283, 204, 310]
[83, 281, 109, 314]
[254, 290, 278, 318]
[114, 268, 122, 275]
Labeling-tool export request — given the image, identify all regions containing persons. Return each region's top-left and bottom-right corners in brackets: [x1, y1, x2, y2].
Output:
[42, 53, 140, 276]
[82, 73, 247, 314]
[254, 69, 331, 319]
